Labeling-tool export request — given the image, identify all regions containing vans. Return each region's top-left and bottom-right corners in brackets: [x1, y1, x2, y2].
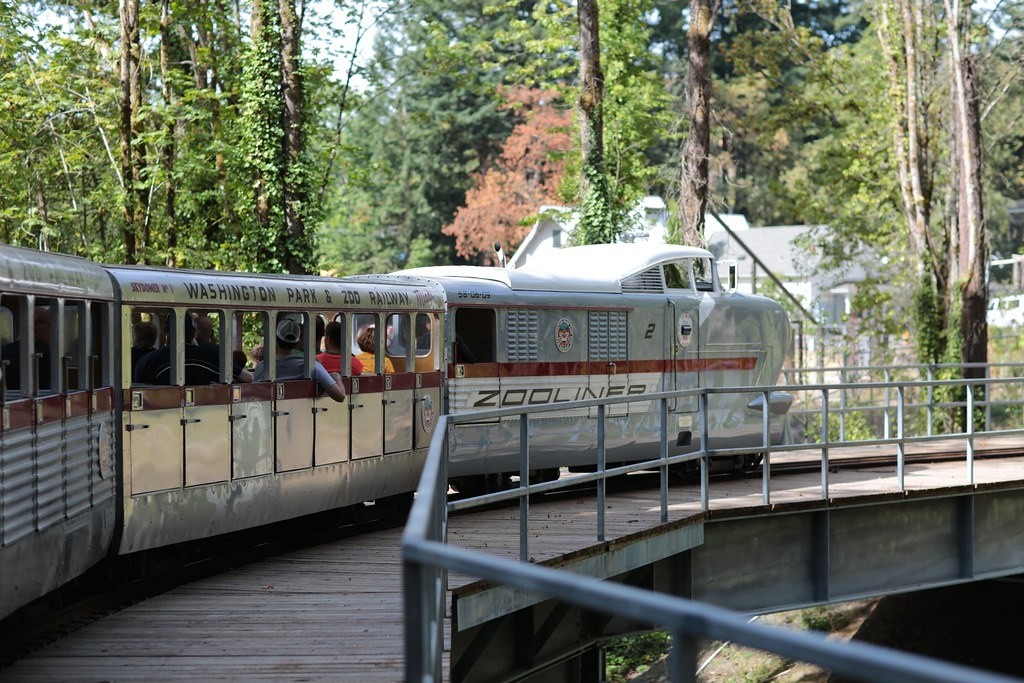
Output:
[986, 295, 1024, 332]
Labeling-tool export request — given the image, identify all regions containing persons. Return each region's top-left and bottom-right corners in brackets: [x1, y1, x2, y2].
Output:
[131, 312, 254, 393]
[417, 316, 476, 364]
[315, 316, 364, 376]
[252, 319, 345, 402]
[355, 328, 394, 373]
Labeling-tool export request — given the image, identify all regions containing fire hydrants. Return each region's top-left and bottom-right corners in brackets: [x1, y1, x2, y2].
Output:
[903, 330, 910, 346]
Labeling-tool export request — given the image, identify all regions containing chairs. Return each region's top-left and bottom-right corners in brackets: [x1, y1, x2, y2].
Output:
[151, 358, 239, 386]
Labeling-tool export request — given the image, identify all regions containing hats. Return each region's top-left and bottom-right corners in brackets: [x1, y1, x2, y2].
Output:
[164, 311, 196, 342]
[276, 319, 300, 343]
[195, 316, 213, 340]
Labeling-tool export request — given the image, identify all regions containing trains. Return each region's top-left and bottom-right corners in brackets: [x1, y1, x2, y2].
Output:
[0, 240, 792, 621]
